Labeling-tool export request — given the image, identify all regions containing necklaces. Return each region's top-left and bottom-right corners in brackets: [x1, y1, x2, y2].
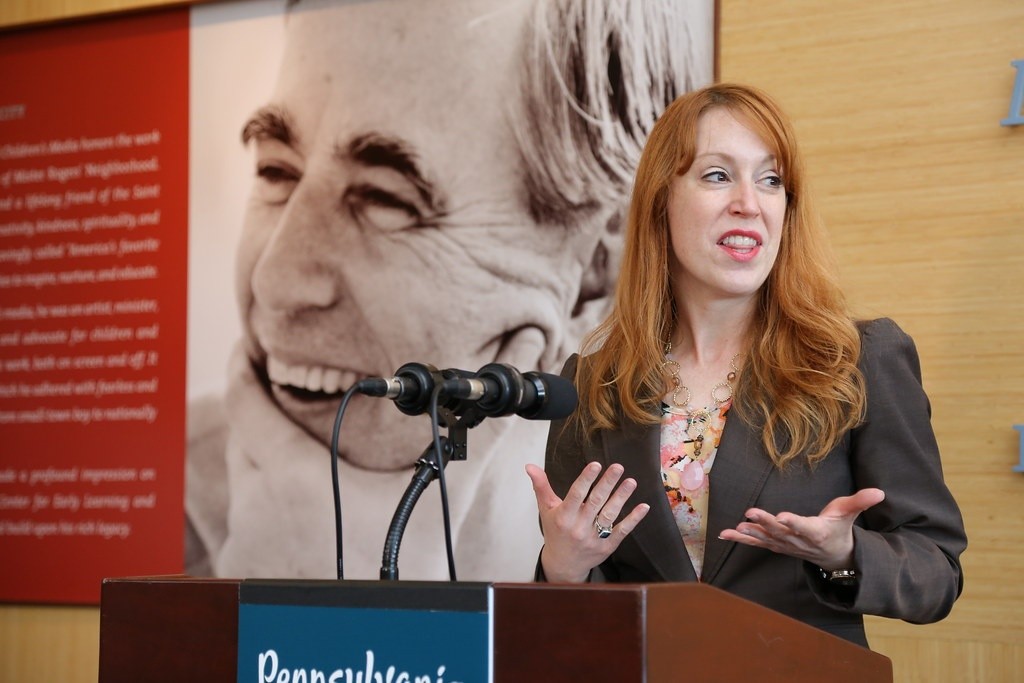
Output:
[660, 320, 752, 492]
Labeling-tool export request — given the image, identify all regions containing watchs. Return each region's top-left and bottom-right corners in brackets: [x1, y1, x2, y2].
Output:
[819, 567, 856, 586]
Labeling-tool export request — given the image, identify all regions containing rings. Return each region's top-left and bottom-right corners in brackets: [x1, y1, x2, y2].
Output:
[595, 516, 612, 538]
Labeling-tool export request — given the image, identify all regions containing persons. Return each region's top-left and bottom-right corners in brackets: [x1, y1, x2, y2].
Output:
[185, 0, 700, 582]
[524, 80, 968, 649]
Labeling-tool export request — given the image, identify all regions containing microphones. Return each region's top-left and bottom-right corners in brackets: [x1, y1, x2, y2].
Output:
[356, 359, 579, 420]
